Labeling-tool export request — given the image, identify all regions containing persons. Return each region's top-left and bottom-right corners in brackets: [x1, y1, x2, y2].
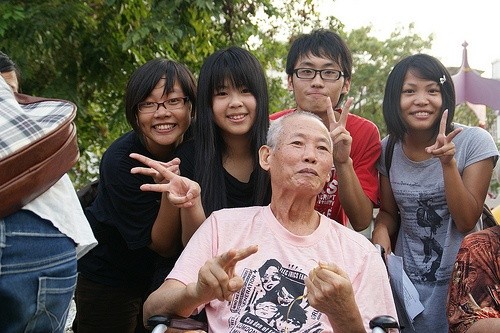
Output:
[143, 111, 400, 333]
[74, 58, 197, 333]
[372, 53, 500, 333]
[447, 225, 500, 333]
[141, 47, 268, 244]
[0, 51, 98, 333]
[268, 29, 383, 232]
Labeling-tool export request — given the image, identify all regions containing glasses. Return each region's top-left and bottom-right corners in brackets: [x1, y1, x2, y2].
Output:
[291, 68, 344, 80]
[137, 96, 190, 113]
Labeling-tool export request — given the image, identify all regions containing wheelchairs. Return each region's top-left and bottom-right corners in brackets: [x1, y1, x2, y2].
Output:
[147, 309, 401, 333]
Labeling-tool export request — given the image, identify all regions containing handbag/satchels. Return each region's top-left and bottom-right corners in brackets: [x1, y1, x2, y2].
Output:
[482, 204, 499, 230]
[0, 73, 80, 219]
